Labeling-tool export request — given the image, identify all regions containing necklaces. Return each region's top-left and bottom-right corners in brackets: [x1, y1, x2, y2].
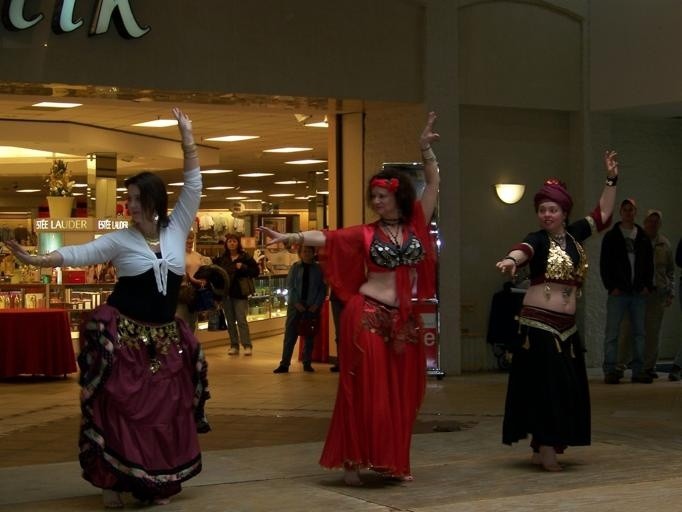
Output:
[551, 232, 569, 248]
[144, 237, 160, 248]
[383, 219, 402, 249]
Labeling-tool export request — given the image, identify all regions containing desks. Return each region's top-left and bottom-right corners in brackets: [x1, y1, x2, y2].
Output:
[0, 309, 77, 380]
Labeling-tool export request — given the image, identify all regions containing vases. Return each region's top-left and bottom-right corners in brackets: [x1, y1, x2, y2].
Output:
[47, 196, 75, 220]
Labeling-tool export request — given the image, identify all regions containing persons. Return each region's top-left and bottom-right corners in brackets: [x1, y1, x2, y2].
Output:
[176, 230, 213, 334]
[486, 270, 529, 371]
[218, 234, 260, 356]
[599, 197, 653, 385]
[256, 111, 441, 485]
[330, 287, 347, 372]
[272, 245, 327, 374]
[643, 208, 673, 379]
[495, 147, 619, 472]
[668, 238, 682, 382]
[2, 107, 203, 509]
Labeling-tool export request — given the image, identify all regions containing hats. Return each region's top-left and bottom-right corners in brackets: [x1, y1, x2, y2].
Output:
[644, 209, 662, 221]
[620, 198, 636, 207]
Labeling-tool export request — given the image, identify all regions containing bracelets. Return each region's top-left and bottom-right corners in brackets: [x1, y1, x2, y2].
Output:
[504, 256, 519, 264]
[605, 177, 618, 187]
[283, 230, 305, 246]
[180, 141, 199, 159]
[420, 143, 438, 165]
[32, 254, 53, 267]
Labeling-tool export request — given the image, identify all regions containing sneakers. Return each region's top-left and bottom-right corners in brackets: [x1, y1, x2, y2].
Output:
[228, 347, 238, 354]
[304, 365, 314, 372]
[273, 366, 289, 373]
[604, 368, 682, 384]
[243, 346, 252, 355]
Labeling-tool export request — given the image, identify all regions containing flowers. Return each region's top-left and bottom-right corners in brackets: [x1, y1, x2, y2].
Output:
[42, 159, 76, 196]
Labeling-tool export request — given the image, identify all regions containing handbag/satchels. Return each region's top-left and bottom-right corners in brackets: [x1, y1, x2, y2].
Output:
[193, 286, 217, 310]
[235, 276, 256, 297]
[208, 309, 228, 332]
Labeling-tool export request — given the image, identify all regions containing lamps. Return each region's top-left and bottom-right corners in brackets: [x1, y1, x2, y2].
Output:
[495, 183, 526, 204]
